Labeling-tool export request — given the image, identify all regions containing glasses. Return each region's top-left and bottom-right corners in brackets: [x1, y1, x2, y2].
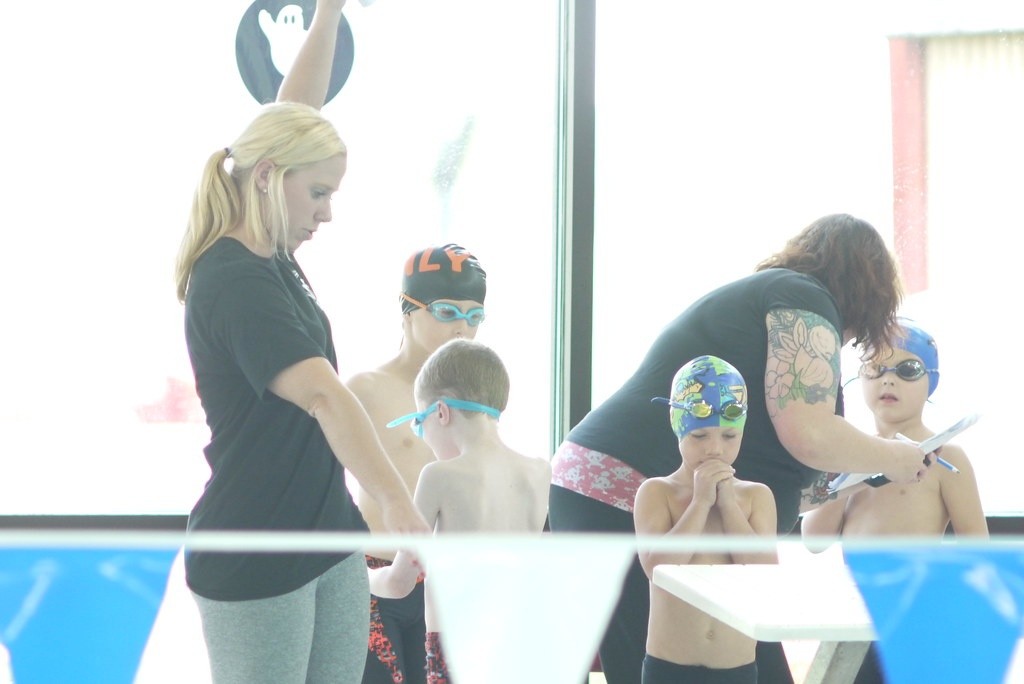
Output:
[668, 399, 748, 420]
[410, 403, 437, 436]
[858, 358, 937, 382]
[401, 291, 484, 327]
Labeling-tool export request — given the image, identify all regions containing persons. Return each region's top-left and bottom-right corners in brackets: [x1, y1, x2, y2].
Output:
[633, 355, 780, 684]
[799, 318, 991, 553]
[550, 212, 900, 684]
[342, 241, 487, 684]
[367, 336, 554, 684]
[173, 0, 434, 684]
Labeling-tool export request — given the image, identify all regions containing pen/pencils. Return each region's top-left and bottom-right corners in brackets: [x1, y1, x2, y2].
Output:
[896, 433, 959, 472]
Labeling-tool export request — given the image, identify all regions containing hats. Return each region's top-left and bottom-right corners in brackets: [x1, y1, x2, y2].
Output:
[854, 315, 940, 396]
[399, 243, 488, 312]
[670, 355, 748, 439]
